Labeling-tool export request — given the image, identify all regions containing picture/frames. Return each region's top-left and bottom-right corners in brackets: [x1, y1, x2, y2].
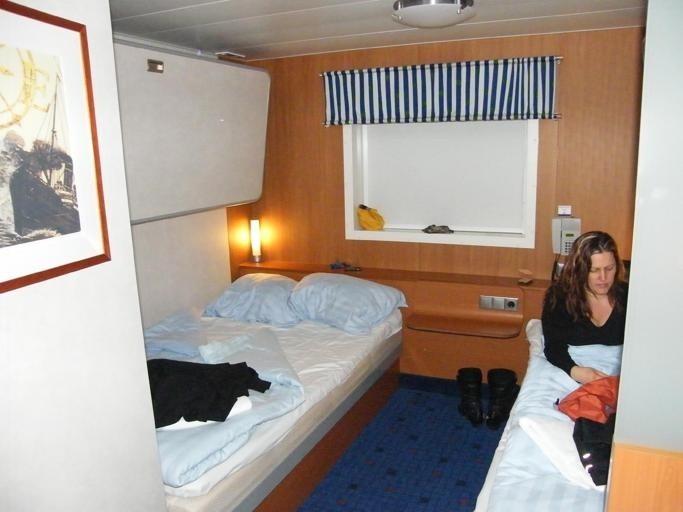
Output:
[0, 0, 112, 292]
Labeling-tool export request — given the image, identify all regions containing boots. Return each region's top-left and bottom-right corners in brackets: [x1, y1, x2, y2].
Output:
[455, 367, 484, 426]
[486, 368, 520, 430]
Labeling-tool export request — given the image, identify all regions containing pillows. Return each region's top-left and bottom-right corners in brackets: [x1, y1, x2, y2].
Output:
[203, 272, 310, 331]
[524, 315, 549, 358]
[291, 271, 417, 338]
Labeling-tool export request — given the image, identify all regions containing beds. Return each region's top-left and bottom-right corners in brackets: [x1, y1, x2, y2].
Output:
[140, 282, 397, 512]
[470, 312, 626, 511]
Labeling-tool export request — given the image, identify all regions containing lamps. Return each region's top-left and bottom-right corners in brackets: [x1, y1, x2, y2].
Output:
[391, 1, 476, 31]
[246, 203, 268, 266]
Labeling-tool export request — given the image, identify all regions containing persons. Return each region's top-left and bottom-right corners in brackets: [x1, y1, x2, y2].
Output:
[540, 230, 628, 384]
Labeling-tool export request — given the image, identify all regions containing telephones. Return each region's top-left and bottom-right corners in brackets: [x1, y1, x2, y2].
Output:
[552, 218, 581, 256]
